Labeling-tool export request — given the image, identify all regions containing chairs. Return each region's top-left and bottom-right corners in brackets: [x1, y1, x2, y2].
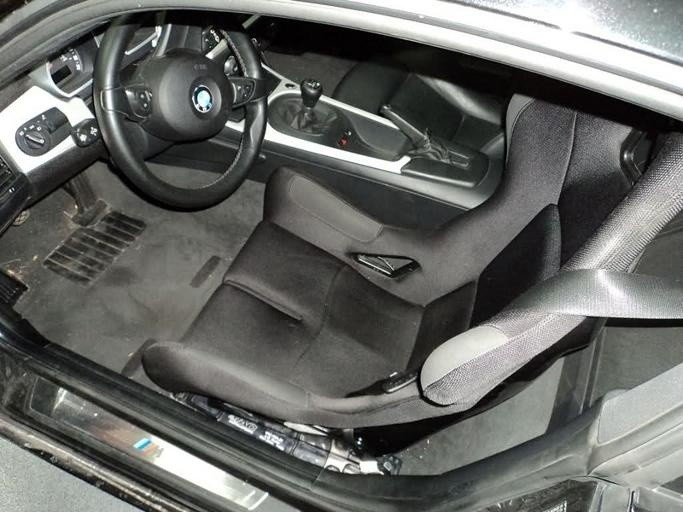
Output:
[140, 90, 683, 459]
